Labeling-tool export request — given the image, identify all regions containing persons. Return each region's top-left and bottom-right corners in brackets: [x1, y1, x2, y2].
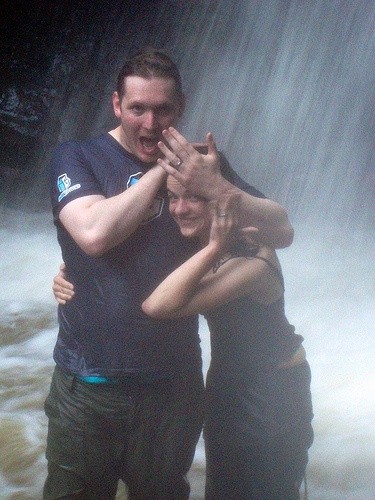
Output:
[41, 47, 295, 500]
[52, 145, 315, 500]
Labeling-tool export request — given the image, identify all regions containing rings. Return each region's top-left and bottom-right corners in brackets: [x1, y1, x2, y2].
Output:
[218, 213, 227, 217]
[175, 159, 184, 168]
